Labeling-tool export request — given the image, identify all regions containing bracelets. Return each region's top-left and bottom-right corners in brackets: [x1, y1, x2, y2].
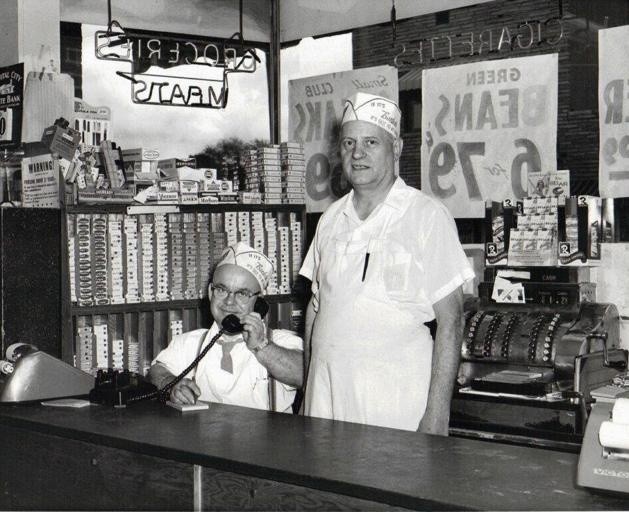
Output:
[251, 340, 271, 354]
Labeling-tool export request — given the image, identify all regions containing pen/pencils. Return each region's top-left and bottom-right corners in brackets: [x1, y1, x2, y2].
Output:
[362, 253, 369, 281]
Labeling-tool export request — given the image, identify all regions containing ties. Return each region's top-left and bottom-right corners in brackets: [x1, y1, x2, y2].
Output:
[213, 337, 242, 373]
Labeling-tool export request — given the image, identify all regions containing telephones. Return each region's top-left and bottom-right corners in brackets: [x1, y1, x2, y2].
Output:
[222, 297, 269, 333]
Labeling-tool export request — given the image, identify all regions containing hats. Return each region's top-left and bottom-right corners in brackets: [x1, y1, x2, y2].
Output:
[216, 243, 274, 292]
[341, 92, 401, 138]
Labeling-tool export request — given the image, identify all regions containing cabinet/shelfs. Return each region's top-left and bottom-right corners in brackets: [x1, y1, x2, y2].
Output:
[0, 203, 308, 377]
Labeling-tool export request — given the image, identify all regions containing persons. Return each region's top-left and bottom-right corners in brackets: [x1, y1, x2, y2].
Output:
[147, 240, 305, 412]
[290, 89, 477, 437]
